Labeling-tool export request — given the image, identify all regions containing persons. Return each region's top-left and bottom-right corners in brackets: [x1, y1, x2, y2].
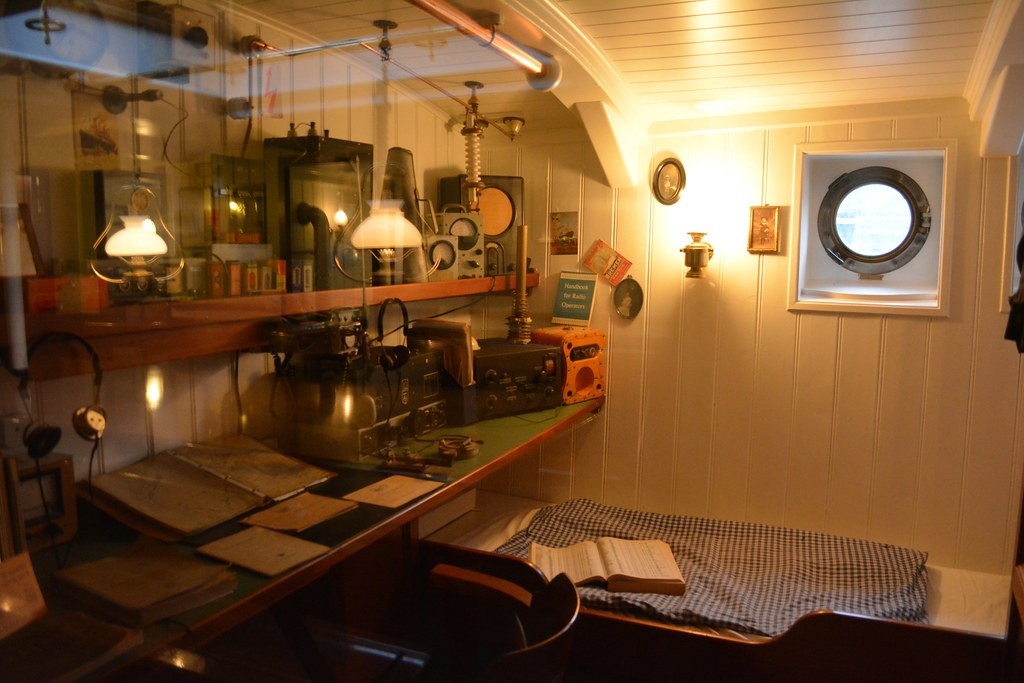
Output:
[758, 215, 771, 246]
[617, 291, 635, 317]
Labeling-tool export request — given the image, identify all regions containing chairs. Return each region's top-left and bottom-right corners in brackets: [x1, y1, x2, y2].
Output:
[307, 539, 582, 683]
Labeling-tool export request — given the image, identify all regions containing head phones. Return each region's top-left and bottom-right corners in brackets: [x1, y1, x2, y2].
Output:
[18, 332, 106, 460]
[377, 297, 411, 372]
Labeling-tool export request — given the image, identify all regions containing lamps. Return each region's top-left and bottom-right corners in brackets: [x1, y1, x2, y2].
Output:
[680, 230, 715, 280]
[333, 160, 441, 281]
[91, 0, 184, 303]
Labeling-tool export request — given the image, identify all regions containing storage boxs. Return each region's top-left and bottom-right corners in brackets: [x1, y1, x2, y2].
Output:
[388, 487, 477, 541]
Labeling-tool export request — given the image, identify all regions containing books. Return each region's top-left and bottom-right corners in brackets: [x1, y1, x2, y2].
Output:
[0, 612, 144, 683]
[81, 430, 339, 537]
[49, 532, 242, 629]
[528, 536, 686, 597]
[552, 270, 598, 327]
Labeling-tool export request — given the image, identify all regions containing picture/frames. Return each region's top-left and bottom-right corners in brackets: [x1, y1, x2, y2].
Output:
[652, 157, 686, 205]
[614, 276, 643, 319]
[746, 204, 782, 254]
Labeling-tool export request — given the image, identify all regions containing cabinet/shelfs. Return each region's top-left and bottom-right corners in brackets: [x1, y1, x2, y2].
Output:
[0, 272, 606, 683]
